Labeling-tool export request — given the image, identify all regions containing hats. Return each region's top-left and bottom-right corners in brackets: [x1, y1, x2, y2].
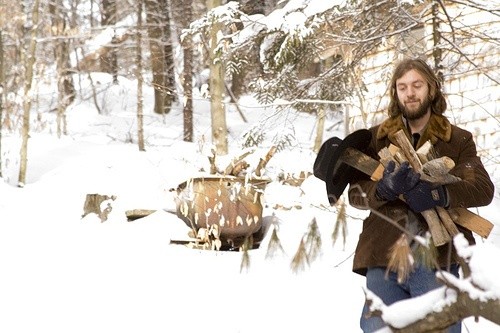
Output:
[314, 129, 372, 206]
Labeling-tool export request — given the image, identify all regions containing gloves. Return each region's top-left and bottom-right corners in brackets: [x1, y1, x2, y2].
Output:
[402, 179, 447, 214]
[374, 159, 421, 203]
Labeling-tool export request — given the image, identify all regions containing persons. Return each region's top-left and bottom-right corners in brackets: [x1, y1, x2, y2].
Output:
[348, 58, 495, 333]
